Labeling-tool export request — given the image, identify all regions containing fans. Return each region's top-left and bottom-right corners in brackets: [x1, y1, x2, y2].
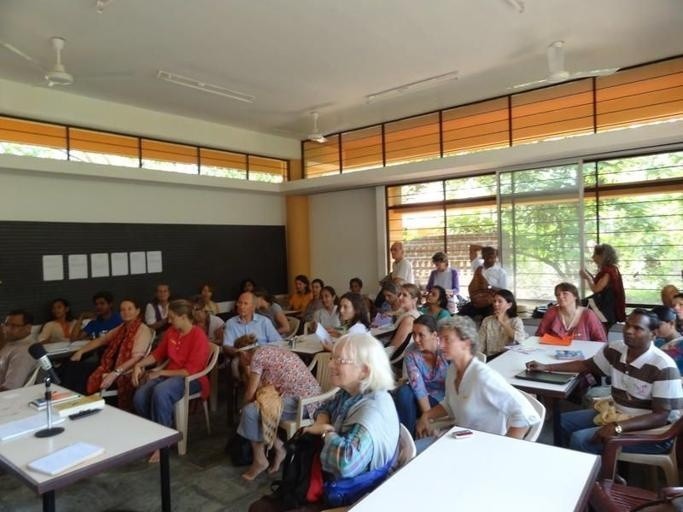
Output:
[1, 33, 133, 92]
[271, 111, 343, 145]
[501, 40, 620, 93]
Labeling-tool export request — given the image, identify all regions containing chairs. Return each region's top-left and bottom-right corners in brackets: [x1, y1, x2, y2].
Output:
[591, 417, 683, 511]
[1, 292, 683, 484]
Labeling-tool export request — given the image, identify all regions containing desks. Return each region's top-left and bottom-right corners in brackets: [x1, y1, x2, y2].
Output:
[0, 382, 183, 509]
[349, 425, 604, 512]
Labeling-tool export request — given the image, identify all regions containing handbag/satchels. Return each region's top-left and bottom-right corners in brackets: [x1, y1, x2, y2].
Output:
[468, 265, 496, 309]
[580, 271, 618, 329]
[532, 303, 554, 319]
[305, 453, 325, 503]
[224, 422, 288, 467]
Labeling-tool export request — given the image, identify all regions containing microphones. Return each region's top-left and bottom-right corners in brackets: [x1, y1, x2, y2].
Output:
[28, 342, 60, 385]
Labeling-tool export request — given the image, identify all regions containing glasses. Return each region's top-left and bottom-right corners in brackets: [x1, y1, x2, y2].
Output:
[327, 356, 354, 366]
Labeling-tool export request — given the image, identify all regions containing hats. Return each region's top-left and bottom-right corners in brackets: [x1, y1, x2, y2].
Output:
[652, 305, 679, 321]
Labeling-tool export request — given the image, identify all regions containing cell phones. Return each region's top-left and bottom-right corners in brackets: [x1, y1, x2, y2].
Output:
[454, 429, 473, 438]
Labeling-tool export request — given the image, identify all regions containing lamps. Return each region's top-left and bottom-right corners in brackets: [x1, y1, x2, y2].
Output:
[155, 69, 255, 106]
[366, 70, 459, 106]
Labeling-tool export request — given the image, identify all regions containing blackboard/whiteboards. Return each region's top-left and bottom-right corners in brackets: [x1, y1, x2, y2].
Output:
[0, 221, 289, 327]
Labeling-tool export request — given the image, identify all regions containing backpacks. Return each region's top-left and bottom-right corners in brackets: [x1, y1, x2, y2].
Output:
[271, 428, 314, 510]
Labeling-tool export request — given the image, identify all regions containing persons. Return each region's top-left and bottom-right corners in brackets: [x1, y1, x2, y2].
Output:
[0, 241, 682, 512]
